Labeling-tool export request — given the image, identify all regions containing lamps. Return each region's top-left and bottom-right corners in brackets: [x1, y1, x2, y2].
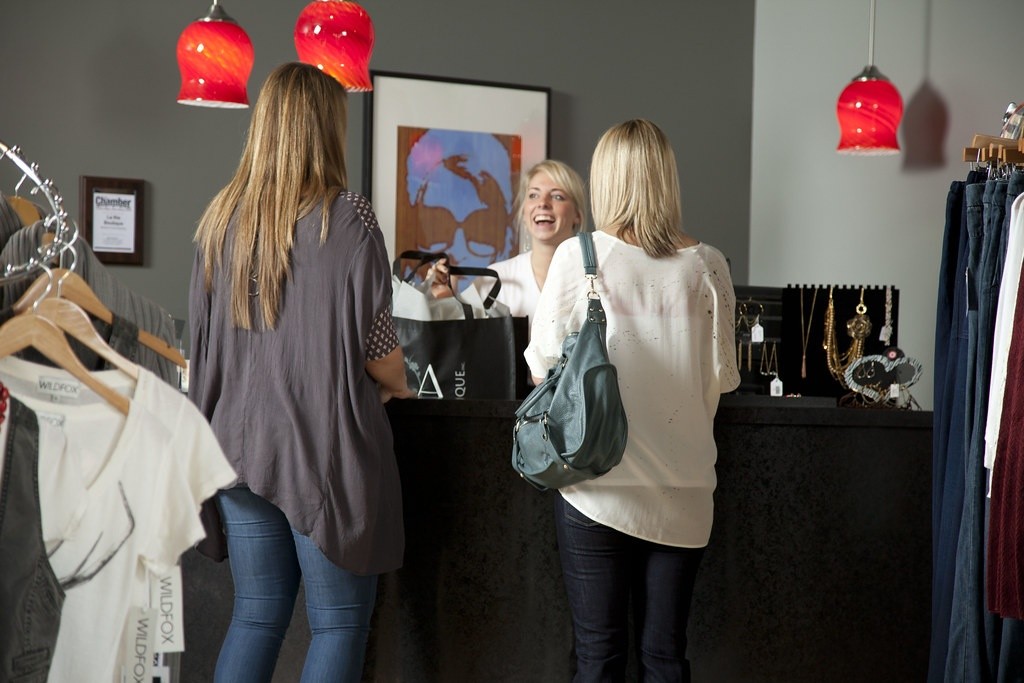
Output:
[293, 0, 375, 93]
[178, 0, 256, 109]
[836, 0, 902, 158]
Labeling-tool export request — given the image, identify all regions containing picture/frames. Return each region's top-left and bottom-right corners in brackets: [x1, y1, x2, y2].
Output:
[362, 70, 553, 299]
[78, 175, 145, 266]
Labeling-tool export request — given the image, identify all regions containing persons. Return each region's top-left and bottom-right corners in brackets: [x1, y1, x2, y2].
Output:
[188, 65, 420, 683]
[424, 160, 591, 382]
[523, 119, 742, 683]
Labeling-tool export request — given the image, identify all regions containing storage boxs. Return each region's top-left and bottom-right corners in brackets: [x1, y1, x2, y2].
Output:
[738, 397, 837, 408]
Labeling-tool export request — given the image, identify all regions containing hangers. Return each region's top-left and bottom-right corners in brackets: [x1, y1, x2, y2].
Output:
[962, 120, 1024, 179]
[0, 148, 187, 417]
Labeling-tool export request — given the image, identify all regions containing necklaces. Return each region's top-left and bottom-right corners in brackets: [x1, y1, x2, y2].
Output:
[793, 286, 892, 389]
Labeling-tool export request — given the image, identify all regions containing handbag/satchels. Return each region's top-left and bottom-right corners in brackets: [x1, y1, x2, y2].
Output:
[510, 232, 628, 493]
[389, 251, 530, 401]
